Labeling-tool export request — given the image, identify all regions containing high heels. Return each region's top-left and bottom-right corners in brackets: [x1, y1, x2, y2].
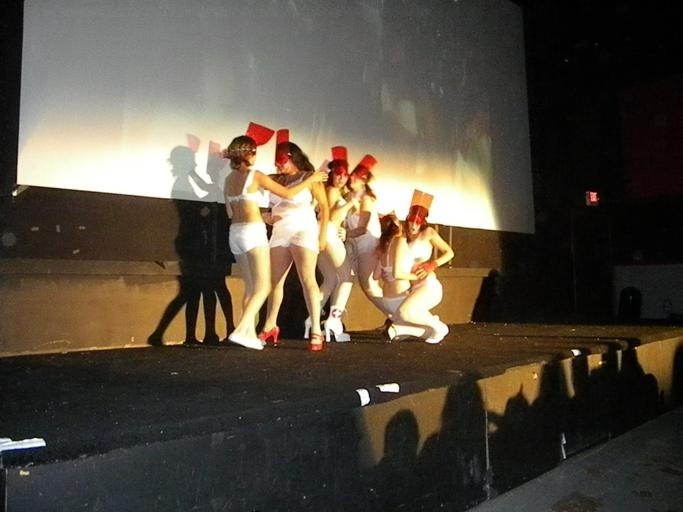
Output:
[258, 326, 280, 348]
[310, 331, 325, 351]
[303, 315, 351, 344]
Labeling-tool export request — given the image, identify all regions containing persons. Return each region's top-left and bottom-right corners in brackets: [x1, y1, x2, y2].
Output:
[304, 157, 367, 343]
[222, 134, 329, 352]
[259, 141, 330, 351]
[338, 163, 393, 332]
[398, 205, 455, 345]
[373, 214, 430, 343]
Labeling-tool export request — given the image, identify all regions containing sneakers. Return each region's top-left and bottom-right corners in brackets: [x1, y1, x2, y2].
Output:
[229, 332, 264, 351]
[426, 326, 450, 344]
